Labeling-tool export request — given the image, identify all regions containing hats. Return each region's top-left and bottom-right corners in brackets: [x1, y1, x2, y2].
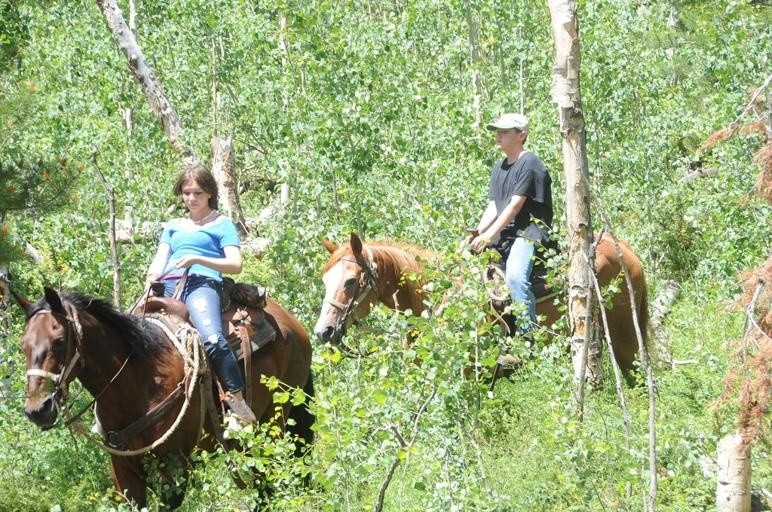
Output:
[486, 112, 529, 135]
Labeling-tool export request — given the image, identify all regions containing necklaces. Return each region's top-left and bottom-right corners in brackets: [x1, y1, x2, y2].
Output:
[186, 208, 216, 223]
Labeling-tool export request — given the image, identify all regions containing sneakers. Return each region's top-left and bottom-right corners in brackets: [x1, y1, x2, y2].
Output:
[496, 355, 517, 368]
[223, 399, 254, 439]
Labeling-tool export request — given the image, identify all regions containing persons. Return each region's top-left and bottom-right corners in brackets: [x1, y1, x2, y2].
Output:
[465, 113, 553, 368]
[90, 163, 256, 441]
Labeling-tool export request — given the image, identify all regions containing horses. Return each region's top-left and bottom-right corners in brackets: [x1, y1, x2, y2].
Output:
[313, 230, 653, 391]
[8, 279, 312, 512]
